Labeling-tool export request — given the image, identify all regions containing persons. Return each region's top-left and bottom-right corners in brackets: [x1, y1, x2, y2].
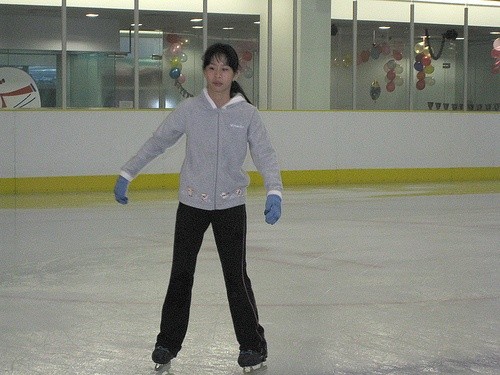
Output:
[113, 42, 282, 374]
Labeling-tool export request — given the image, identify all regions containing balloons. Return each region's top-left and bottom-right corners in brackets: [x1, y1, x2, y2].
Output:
[166, 33, 187, 84]
[235, 40, 254, 78]
[491, 37, 500, 76]
[414, 41, 436, 91]
[338, 40, 404, 92]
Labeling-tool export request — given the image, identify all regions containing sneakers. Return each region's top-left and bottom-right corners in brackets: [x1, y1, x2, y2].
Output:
[238, 349, 268, 375]
[152, 345, 173, 371]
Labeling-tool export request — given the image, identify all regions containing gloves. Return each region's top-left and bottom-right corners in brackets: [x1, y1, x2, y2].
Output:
[264, 194, 281, 225]
[114, 175, 128, 204]
